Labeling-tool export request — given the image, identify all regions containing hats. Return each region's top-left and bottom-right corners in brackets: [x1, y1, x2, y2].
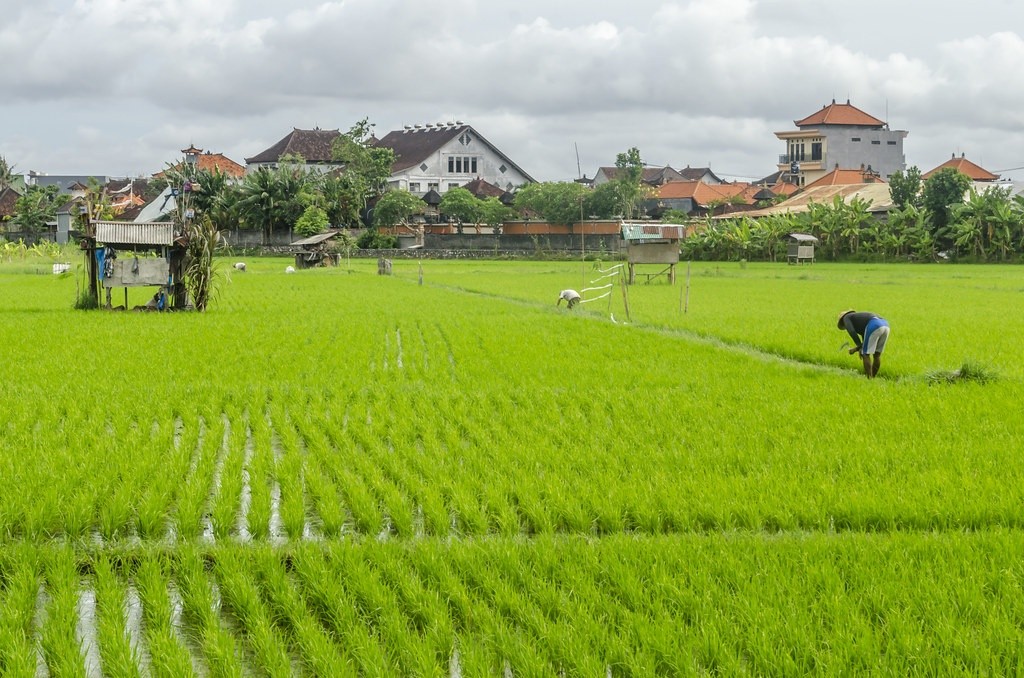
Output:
[838, 310, 855, 330]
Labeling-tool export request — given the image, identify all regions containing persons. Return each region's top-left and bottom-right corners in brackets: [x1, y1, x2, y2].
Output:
[146, 293, 160, 307]
[557, 290, 580, 309]
[837, 310, 890, 379]
[233, 262, 246, 272]
